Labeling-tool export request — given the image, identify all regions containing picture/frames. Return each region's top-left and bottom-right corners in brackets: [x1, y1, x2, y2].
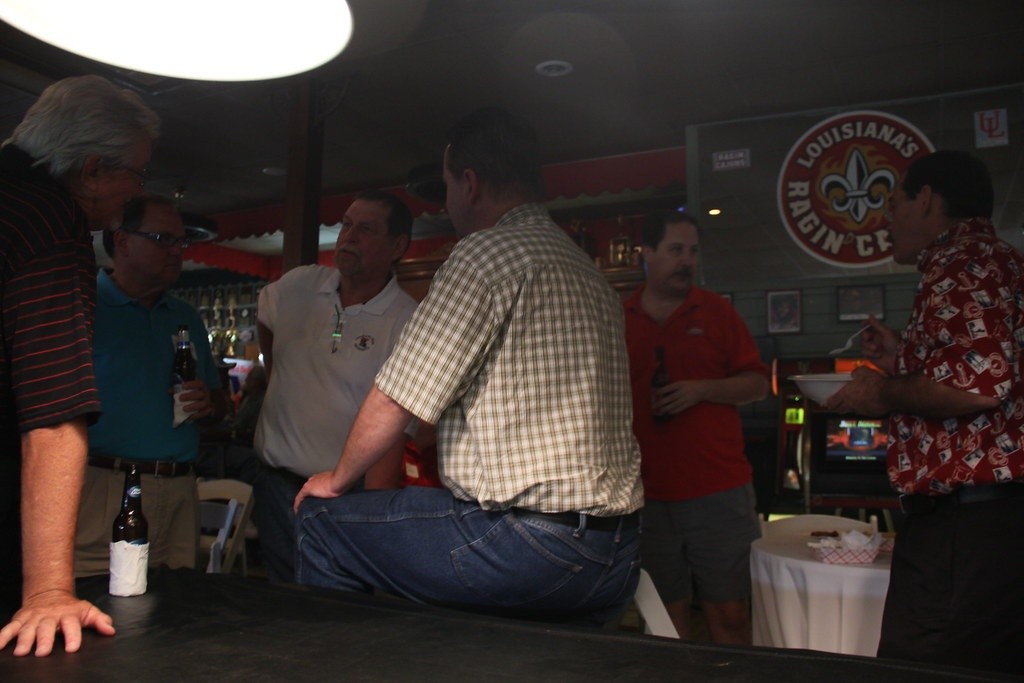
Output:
[764, 289, 802, 335]
[835, 283, 885, 322]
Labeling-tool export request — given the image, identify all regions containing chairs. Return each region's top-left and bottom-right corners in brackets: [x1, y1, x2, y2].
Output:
[756, 510, 879, 534]
[196, 478, 254, 577]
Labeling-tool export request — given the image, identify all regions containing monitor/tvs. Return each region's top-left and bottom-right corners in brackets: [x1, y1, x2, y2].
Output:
[808, 410, 899, 496]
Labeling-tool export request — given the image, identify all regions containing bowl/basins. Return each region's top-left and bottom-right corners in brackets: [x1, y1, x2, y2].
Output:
[786, 373, 855, 405]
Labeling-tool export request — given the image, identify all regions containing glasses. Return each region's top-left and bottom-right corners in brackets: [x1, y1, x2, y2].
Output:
[328, 304, 347, 353]
[120, 226, 195, 251]
[123, 161, 148, 192]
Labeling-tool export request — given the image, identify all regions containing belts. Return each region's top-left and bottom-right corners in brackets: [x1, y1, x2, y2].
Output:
[898, 480, 1024, 515]
[87, 454, 196, 479]
[513, 508, 643, 534]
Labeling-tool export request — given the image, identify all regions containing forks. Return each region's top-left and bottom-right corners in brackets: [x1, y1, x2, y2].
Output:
[829, 323, 873, 356]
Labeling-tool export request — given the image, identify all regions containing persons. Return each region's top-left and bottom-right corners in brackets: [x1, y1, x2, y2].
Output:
[65, 195, 224, 582]
[621, 209, 769, 652]
[0, 75, 160, 657]
[243, 190, 428, 581]
[288, 109, 644, 626]
[233, 366, 267, 448]
[821, 150, 1023, 682]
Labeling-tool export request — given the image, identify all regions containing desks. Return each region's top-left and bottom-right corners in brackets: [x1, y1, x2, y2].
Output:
[0, 574, 1018, 683]
[750, 532, 894, 657]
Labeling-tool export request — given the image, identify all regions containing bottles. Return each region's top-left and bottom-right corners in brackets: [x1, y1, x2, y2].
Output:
[109, 463, 150, 597]
[609, 214, 631, 267]
[171, 324, 197, 394]
[650, 346, 673, 413]
